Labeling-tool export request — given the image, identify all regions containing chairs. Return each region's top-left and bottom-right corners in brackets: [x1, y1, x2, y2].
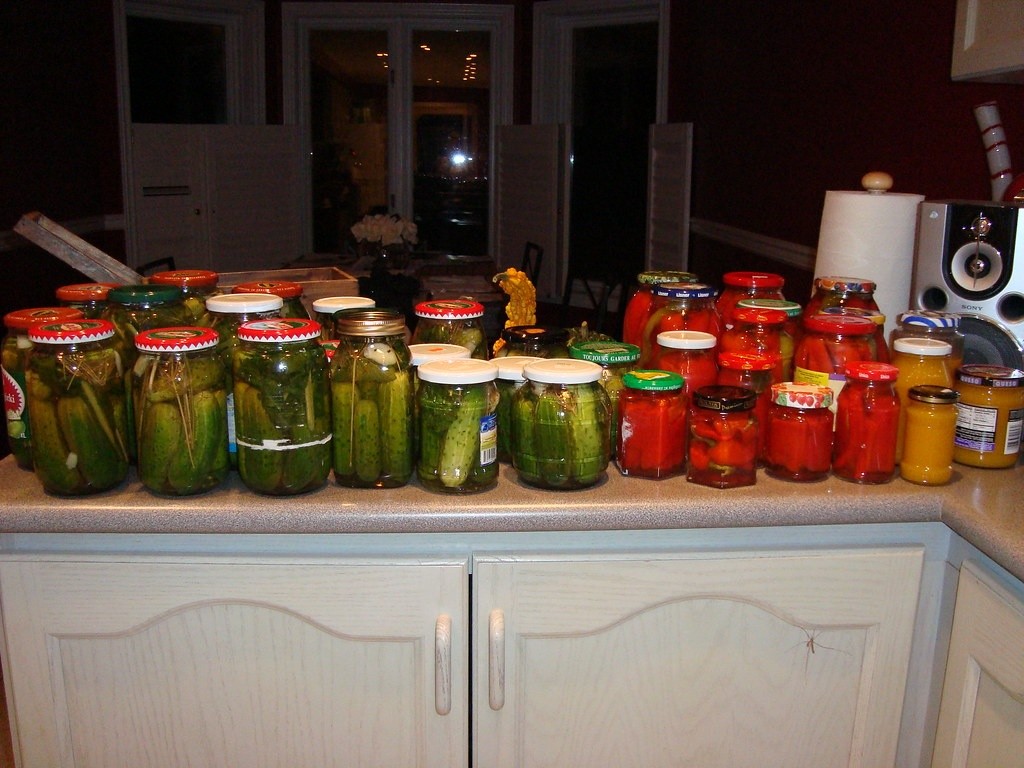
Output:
[136, 257, 176, 276]
[522, 240, 544, 285]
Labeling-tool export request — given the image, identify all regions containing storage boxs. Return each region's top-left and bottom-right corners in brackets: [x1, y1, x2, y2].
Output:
[216, 268, 359, 321]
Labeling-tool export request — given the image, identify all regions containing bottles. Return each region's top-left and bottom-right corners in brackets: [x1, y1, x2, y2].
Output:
[0, 266, 1022, 499]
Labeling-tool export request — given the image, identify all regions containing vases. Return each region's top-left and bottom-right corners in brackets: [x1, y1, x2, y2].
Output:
[359, 240, 409, 269]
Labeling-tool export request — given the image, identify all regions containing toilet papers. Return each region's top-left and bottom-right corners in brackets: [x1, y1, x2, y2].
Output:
[811, 190, 926, 360]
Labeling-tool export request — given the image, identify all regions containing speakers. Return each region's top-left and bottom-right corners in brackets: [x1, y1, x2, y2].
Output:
[912, 200, 1024, 378]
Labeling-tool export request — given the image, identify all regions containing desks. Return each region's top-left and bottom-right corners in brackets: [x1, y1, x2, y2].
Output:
[275, 255, 497, 306]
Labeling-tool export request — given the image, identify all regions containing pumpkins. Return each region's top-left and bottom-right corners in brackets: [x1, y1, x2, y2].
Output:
[490, 268, 538, 356]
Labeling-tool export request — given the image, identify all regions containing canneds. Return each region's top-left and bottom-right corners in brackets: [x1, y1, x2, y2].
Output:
[0, 269, 1024, 498]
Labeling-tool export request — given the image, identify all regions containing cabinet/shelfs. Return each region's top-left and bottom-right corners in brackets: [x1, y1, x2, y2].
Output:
[0, 521, 1024, 768]
[951, 0, 1024, 84]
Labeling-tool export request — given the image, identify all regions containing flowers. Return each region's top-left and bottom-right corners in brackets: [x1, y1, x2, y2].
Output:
[350, 214, 417, 244]
